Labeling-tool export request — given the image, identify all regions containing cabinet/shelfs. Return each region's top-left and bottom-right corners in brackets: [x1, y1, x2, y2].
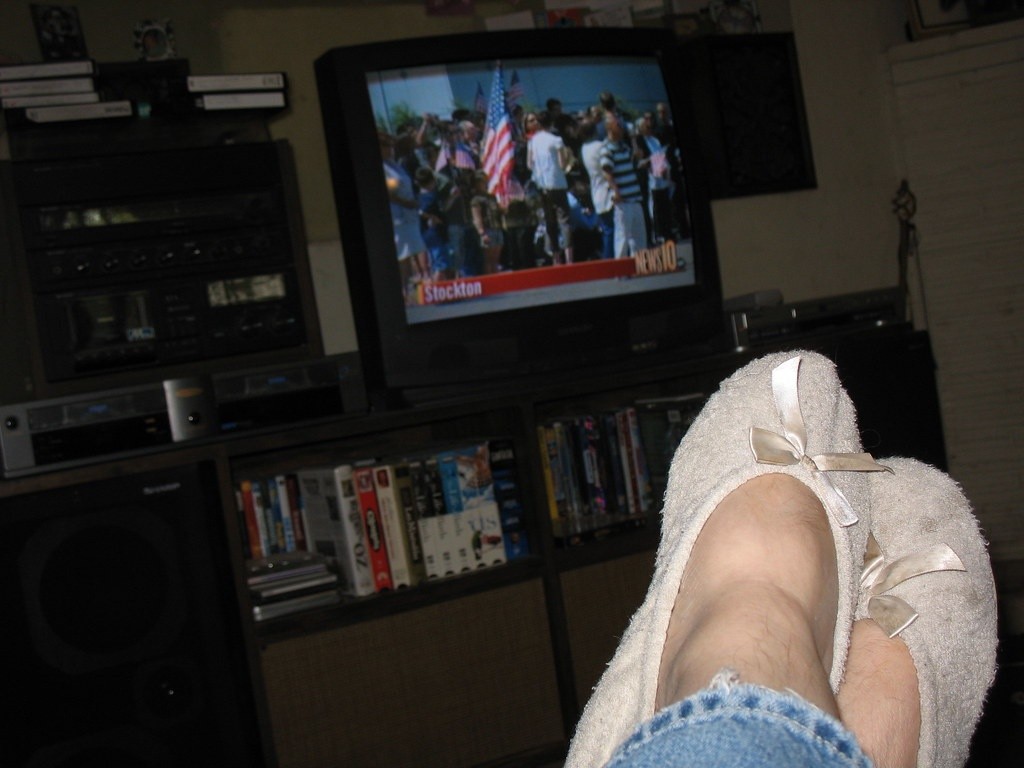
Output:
[216, 367, 731, 768]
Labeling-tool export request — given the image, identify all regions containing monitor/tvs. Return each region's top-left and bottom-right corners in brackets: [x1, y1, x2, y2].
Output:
[315, 28, 730, 404]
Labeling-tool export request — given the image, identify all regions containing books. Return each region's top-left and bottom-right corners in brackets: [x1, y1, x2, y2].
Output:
[228, 437, 528, 623]
[185, 71, 287, 112]
[535, 405, 705, 539]
[0, 60, 133, 124]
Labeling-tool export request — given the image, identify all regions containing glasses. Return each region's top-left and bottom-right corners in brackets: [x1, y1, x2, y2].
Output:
[527, 117, 540, 122]
[447, 129, 461, 135]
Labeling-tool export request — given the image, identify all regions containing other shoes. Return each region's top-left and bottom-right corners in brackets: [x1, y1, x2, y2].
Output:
[563, 348, 870, 768]
[853, 455, 999, 768]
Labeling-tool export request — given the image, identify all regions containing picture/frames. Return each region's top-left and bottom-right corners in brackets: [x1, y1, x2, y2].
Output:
[904, 0, 973, 42]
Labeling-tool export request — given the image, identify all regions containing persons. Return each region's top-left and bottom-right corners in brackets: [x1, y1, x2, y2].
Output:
[562, 349, 998, 768]
[369, 91, 692, 305]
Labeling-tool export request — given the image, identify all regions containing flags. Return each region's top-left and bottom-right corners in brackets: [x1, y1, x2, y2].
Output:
[473, 60, 525, 211]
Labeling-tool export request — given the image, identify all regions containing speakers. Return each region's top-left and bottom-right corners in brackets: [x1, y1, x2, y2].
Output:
[680, 30, 818, 201]
